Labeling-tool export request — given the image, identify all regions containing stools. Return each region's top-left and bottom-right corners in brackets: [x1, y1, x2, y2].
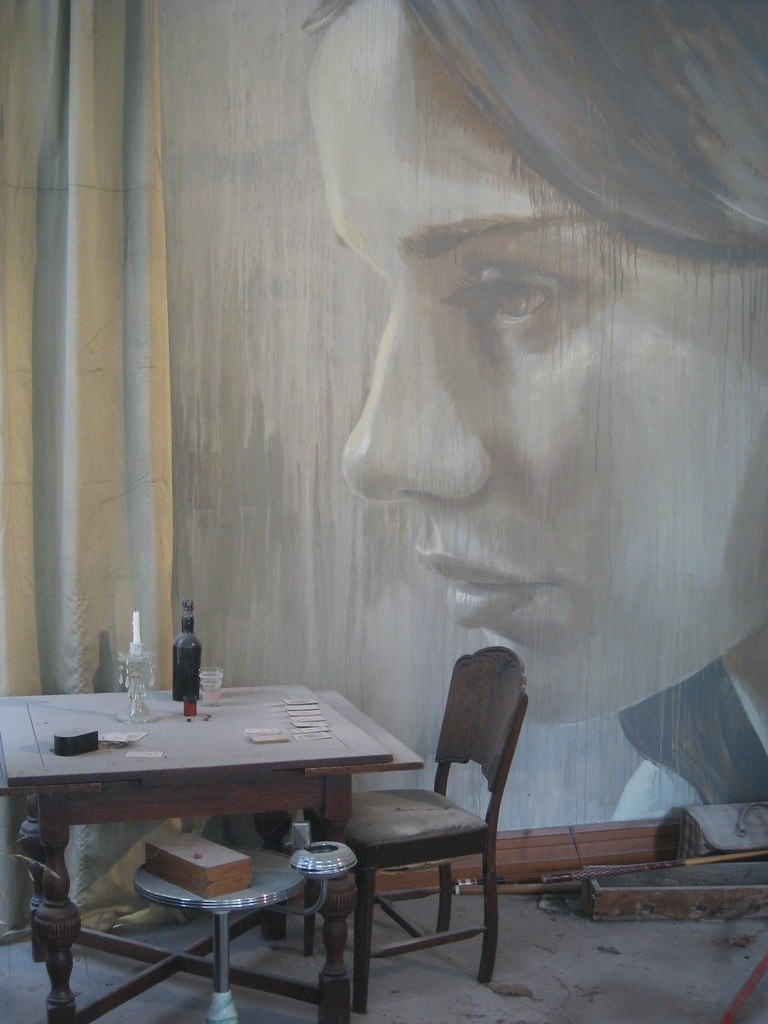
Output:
[132, 847, 306, 1024]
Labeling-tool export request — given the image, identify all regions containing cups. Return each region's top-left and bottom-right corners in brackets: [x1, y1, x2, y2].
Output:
[183, 694, 197, 716]
[199, 664, 223, 706]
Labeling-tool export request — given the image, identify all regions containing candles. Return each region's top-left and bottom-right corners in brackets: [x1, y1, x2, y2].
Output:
[132, 608, 140, 643]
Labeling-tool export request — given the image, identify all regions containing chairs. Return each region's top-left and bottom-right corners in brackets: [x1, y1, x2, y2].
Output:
[303, 645, 530, 1014]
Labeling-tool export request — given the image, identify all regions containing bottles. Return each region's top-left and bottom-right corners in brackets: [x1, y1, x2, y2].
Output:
[171, 599, 202, 702]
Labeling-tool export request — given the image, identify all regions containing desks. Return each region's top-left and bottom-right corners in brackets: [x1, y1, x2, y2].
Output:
[0, 685, 424, 1024]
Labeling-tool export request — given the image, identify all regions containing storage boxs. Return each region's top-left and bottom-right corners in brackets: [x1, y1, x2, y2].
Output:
[145, 832, 252, 898]
